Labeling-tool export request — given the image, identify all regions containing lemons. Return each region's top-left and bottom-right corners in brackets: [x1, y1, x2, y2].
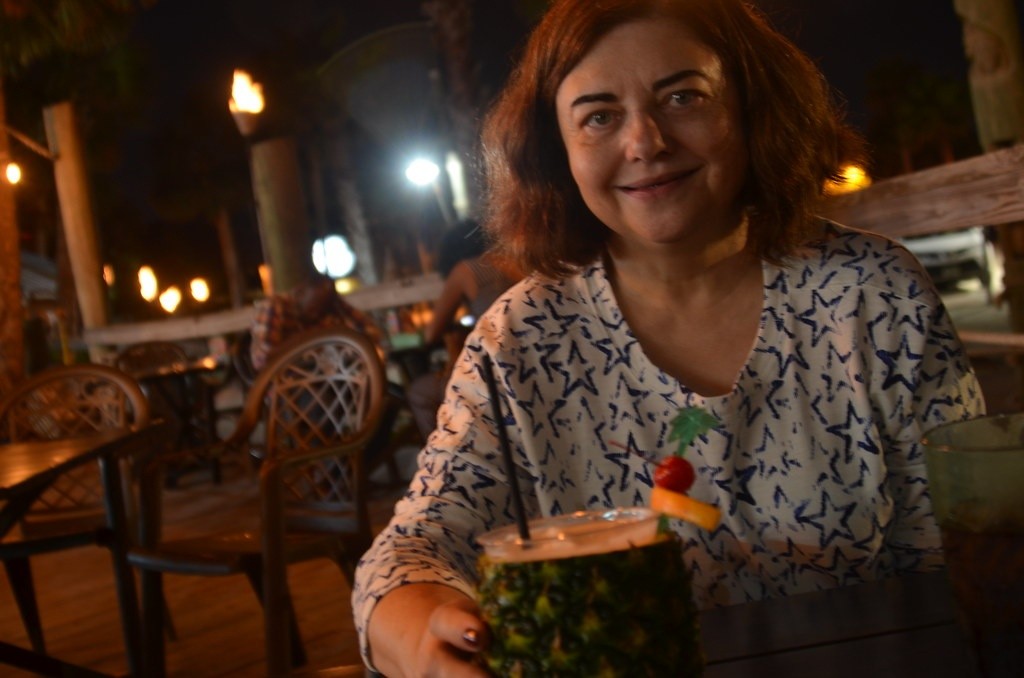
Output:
[647, 484, 719, 531]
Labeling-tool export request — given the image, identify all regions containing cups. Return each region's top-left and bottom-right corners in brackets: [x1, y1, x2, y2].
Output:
[920, 411, 1024, 669]
[470, 504, 675, 678]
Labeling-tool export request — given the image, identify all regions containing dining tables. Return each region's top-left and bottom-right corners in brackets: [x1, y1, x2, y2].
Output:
[301, 505, 1024, 677]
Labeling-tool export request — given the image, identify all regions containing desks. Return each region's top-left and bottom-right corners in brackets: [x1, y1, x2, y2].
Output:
[128, 356, 247, 485]
[358, 326, 448, 390]
[1, 428, 140, 659]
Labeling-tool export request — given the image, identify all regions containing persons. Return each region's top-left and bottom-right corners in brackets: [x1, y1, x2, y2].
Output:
[252, 273, 385, 377]
[407, 219, 522, 444]
[352, 1, 988, 678]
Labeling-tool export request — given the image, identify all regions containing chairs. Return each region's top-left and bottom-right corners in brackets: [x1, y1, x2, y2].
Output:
[0, 419, 175, 678]
[0, 363, 174, 650]
[126, 330, 394, 677]
[111, 339, 210, 451]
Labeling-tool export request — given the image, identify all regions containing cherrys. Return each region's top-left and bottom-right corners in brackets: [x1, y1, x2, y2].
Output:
[653, 457, 696, 494]
